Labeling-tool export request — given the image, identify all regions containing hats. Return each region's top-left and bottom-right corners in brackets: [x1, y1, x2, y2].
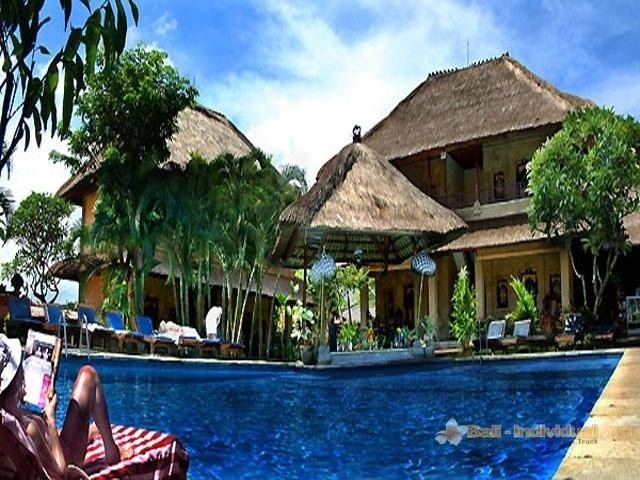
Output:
[0, 333, 22, 393]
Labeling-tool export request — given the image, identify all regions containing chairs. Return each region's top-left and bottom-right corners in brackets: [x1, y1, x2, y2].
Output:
[0, 409, 190, 480]
[555, 313, 585, 351]
[472, 317, 548, 357]
[8, 297, 245, 360]
[591, 314, 619, 349]
